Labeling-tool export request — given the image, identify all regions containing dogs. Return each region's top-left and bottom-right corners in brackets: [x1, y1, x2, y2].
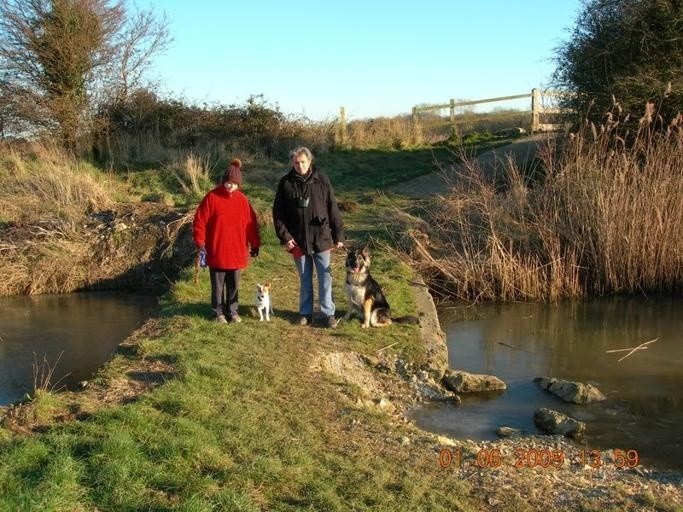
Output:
[255, 283, 275, 322]
[340, 243, 421, 329]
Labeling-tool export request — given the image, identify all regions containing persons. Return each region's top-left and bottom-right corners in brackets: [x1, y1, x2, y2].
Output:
[192, 181, 260, 324]
[273, 146, 344, 329]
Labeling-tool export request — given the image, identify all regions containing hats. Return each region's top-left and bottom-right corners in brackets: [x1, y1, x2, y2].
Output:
[222, 159, 241, 186]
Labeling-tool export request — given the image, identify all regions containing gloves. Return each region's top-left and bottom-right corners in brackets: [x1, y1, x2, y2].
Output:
[250, 247, 259, 257]
[198, 246, 208, 256]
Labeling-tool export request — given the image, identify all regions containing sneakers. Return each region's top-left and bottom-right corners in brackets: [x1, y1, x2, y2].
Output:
[300, 314, 312, 326]
[216, 314, 241, 324]
[322, 315, 337, 329]
